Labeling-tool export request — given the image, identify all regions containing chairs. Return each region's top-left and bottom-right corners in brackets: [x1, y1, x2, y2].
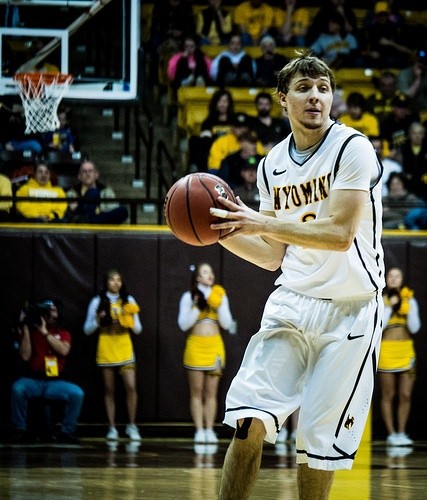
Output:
[177, 47, 398, 130]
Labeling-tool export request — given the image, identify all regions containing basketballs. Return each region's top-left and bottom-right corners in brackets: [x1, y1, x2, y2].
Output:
[163, 173, 237, 247]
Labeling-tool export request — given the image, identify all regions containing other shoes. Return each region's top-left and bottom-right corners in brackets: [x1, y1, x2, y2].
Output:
[205, 428, 217, 442]
[277, 427, 287, 442]
[126, 425, 140, 440]
[107, 428, 118, 440]
[387, 432, 414, 446]
[195, 430, 206, 443]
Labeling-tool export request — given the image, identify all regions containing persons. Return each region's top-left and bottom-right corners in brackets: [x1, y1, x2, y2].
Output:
[84, 267, 141, 440]
[10, 299, 84, 435]
[177, 261, 233, 455]
[0, 0, 427, 229]
[208, 50, 384, 500]
[377, 265, 421, 445]
